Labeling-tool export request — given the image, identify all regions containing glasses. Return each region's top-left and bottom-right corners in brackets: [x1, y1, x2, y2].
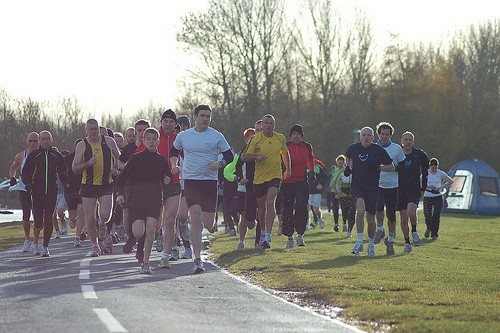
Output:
[27, 139, 39, 143]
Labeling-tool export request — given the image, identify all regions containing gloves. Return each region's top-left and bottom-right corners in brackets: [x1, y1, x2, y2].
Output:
[344, 166, 352, 177]
[10, 177, 17, 185]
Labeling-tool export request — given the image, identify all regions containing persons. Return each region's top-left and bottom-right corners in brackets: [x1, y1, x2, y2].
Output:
[345, 127, 396, 257]
[71, 118, 126, 257]
[9, 131, 40, 251]
[241, 114, 291, 249]
[169, 104, 234, 273]
[419, 157, 454, 240]
[398, 131, 429, 253]
[51, 110, 406, 268]
[20, 130, 69, 257]
[115, 128, 172, 274]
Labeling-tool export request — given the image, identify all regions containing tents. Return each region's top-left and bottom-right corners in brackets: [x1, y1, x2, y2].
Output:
[441, 159, 500, 217]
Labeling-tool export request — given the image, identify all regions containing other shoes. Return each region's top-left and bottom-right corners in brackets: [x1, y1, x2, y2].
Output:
[260, 235, 271, 248]
[225, 229, 236, 235]
[335, 225, 339, 231]
[248, 219, 255, 229]
[425, 229, 438, 240]
[347, 232, 352, 237]
[237, 243, 245, 251]
[343, 226, 347, 232]
[278, 229, 282, 235]
[311, 220, 325, 229]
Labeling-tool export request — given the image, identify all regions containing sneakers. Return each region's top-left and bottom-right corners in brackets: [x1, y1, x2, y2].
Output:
[352, 241, 364, 254]
[367, 245, 375, 257]
[384, 236, 395, 255]
[374, 229, 385, 244]
[18, 218, 205, 274]
[297, 235, 305, 245]
[403, 243, 412, 253]
[412, 230, 420, 246]
[286, 238, 293, 250]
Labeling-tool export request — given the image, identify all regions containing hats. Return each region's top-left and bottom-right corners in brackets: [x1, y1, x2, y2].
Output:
[160, 109, 176, 121]
[241, 128, 255, 137]
[290, 124, 304, 137]
[177, 115, 190, 124]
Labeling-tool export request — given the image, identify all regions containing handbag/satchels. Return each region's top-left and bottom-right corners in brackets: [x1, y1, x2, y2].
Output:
[426, 189, 440, 194]
